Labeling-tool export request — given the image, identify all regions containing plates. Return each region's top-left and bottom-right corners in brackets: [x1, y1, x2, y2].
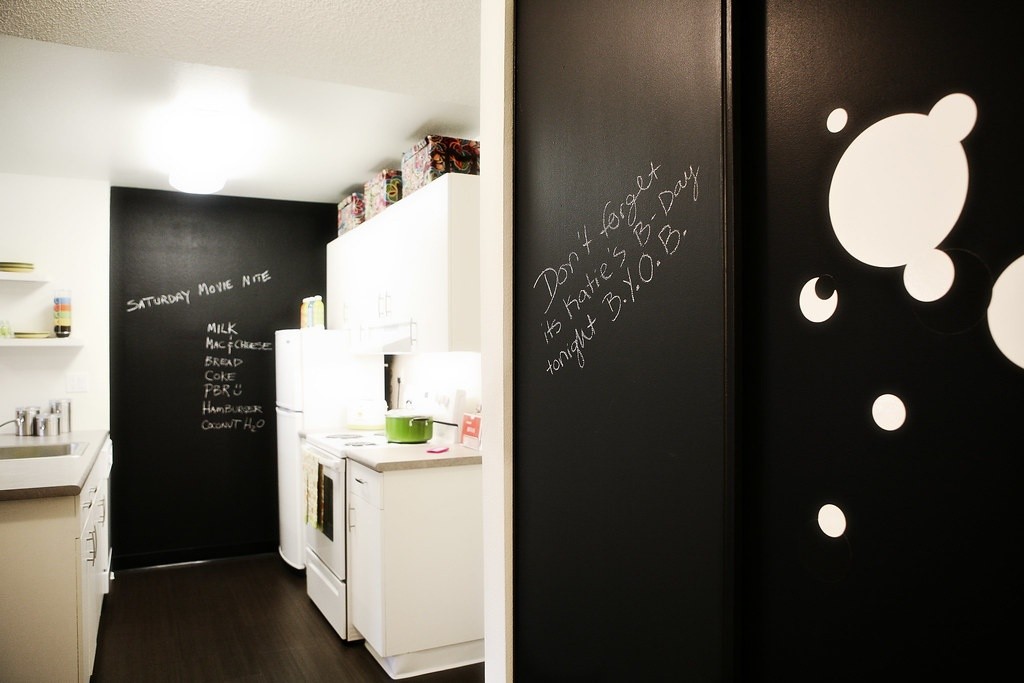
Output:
[14, 333, 51, 337]
[0, 267, 33, 272]
[0, 262, 33, 266]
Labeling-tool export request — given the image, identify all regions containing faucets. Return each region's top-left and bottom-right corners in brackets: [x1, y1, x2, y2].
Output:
[409, 417, 458, 428]
[0, 418, 21, 429]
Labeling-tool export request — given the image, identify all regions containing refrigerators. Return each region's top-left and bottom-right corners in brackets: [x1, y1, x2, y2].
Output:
[275, 329, 371, 569]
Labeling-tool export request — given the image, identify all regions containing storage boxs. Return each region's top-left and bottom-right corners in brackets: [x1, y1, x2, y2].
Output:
[335, 191, 368, 237]
[363, 168, 401, 222]
[401, 133, 481, 198]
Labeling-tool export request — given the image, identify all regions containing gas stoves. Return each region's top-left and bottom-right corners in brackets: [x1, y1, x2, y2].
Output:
[301, 386, 463, 457]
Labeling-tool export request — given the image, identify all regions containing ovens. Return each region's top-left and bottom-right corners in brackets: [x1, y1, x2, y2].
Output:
[298, 442, 363, 642]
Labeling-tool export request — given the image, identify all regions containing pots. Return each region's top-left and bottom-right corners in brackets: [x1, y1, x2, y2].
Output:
[386, 400, 434, 443]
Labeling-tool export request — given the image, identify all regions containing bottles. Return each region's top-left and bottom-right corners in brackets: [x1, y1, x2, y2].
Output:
[299, 294, 326, 329]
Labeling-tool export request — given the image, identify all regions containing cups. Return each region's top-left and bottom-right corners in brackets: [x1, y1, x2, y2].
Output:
[35, 414, 60, 436]
[50, 402, 70, 433]
[53, 297, 72, 337]
[15, 407, 39, 437]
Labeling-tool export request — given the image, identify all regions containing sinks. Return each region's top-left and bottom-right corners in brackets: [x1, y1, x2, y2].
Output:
[0, 441, 88, 462]
[343, 441, 377, 448]
[325, 434, 364, 440]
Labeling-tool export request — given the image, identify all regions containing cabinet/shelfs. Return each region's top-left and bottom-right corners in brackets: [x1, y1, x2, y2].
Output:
[0, 436, 114, 683]
[298, 439, 488, 680]
